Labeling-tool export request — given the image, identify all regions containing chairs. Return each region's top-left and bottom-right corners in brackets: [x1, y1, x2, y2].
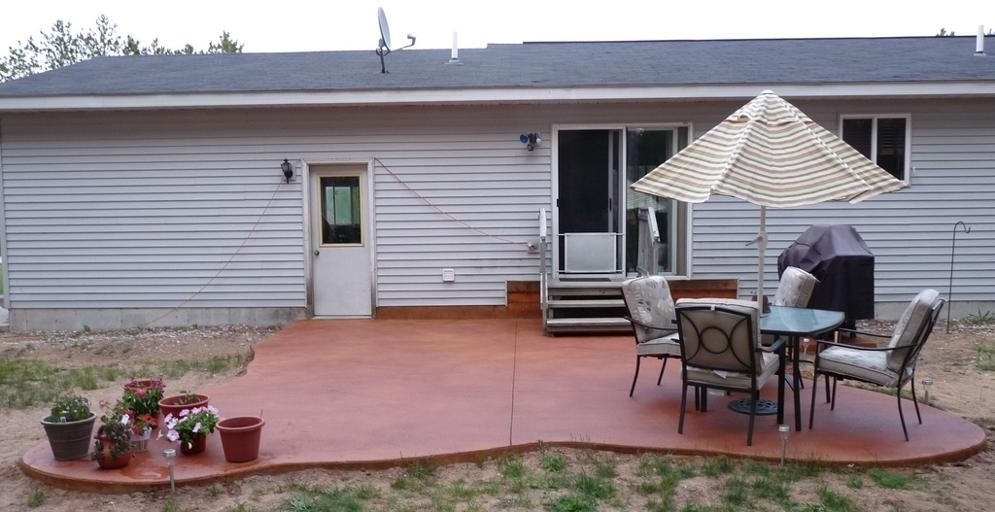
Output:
[619, 275, 700, 409]
[768, 266, 818, 392]
[674, 297, 787, 447]
[807, 288, 946, 442]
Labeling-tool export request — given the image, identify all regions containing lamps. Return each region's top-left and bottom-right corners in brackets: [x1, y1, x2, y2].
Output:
[519, 131, 541, 154]
[281, 157, 293, 186]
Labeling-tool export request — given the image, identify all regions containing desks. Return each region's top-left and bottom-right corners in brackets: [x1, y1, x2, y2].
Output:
[672, 306, 845, 433]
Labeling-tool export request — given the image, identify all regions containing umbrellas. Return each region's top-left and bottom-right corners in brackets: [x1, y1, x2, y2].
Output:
[627, 87, 911, 416]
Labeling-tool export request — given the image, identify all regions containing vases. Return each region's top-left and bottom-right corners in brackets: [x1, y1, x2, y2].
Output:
[39, 377, 265, 471]
